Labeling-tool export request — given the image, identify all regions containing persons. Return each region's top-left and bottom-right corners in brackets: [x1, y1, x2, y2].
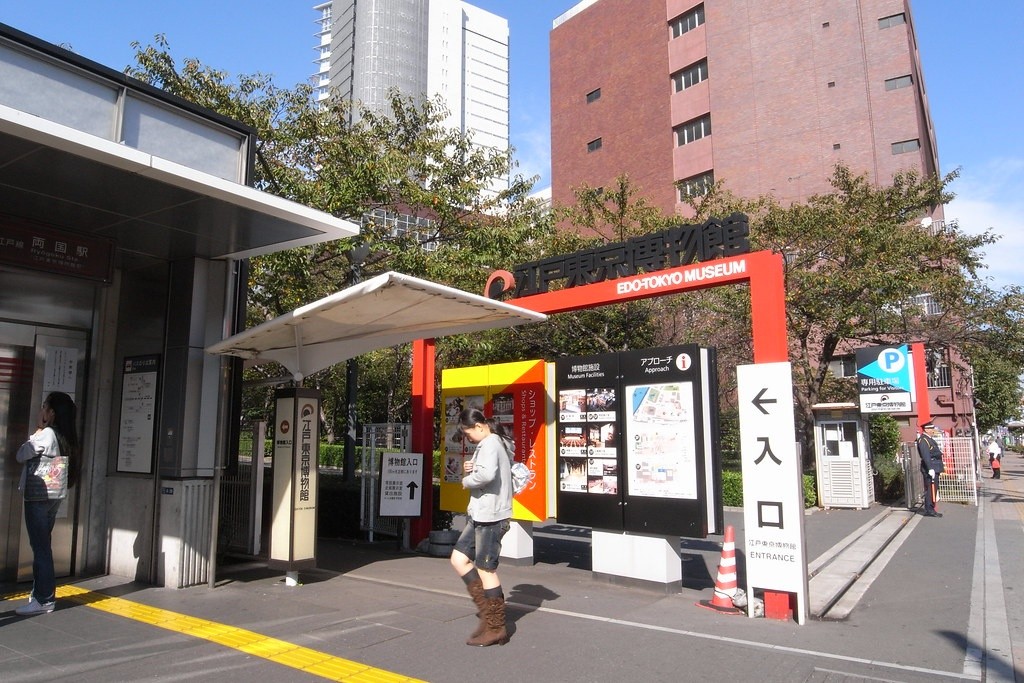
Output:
[449, 408, 516, 647]
[996, 436, 1004, 457]
[986, 437, 1001, 479]
[917, 419, 944, 517]
[15, 392, 82, 615]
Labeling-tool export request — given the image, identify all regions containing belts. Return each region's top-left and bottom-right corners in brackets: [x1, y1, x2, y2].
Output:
[930, 457, 942, 460]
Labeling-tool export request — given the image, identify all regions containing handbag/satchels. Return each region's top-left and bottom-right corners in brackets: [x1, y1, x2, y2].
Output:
[991, 458, 1000, 469]
[23, 436, 70, 501]
[511, 462, 530, 495]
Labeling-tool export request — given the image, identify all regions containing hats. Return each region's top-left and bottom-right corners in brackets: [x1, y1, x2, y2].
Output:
[921, 421, 935, 429]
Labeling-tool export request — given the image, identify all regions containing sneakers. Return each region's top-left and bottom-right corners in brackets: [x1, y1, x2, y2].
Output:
[16, 599, 56, 615]
[28, 593, 34, 603]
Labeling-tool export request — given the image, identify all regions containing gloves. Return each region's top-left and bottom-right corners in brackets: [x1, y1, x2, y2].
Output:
[928, 469, 935, 479]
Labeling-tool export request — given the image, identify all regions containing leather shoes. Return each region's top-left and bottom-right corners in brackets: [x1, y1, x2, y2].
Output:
[931, 512, 943, 517]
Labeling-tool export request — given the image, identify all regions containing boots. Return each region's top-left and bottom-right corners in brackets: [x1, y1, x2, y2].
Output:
[466, 593, 508, 646]
[468, 584, 485, 637]
